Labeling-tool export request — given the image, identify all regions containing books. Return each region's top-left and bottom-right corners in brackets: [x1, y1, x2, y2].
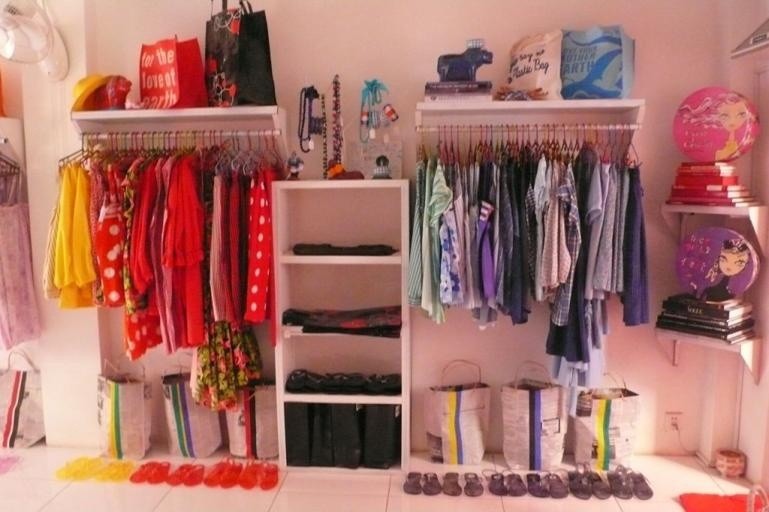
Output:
[664, 159, 763, 208]
[654, 291, 757, 345]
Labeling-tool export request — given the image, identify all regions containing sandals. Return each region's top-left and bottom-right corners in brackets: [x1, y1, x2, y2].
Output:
[57, 457, 281, 491]
[1, 452, 21, 475]
[404, 464, 652, 501]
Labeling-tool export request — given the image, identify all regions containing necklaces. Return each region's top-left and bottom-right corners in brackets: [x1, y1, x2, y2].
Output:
[296, 74, 343, 179]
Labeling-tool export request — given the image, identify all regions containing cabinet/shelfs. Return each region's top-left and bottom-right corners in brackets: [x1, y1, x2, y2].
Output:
[654, 204, 769, 385]
[271, 178, 410, 477]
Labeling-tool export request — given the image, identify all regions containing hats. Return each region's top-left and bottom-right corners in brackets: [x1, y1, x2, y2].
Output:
[68, 73, 125, 124]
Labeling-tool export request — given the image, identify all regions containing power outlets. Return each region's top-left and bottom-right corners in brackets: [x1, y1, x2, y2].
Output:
[664, 412, 683, 433]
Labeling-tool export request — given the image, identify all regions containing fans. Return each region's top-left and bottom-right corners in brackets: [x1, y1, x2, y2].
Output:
[0, 0, 69, 83]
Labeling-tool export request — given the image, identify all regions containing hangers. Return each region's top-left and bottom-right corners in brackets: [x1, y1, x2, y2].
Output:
[58, 129, 285, 176]
[0, 157, 20, 176]
[416, 122, 640, 168]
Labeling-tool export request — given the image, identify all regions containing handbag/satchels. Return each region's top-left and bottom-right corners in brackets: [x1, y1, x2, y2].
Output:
[561, 25, 635, 98]
[508, 29, 566, 99]
[288, 402, 401, 471]
[575, 382, 640, 470]
[223, 378, 278, 459]
[498, 376, 570, 469]
[422, 375, 493, 466]
[98, 374, 155, 459]
[1, 365, 46, 451]
[204, 4, 279, 106]
[137, 34, 208, 109]
[160, 370, 222, 458]
